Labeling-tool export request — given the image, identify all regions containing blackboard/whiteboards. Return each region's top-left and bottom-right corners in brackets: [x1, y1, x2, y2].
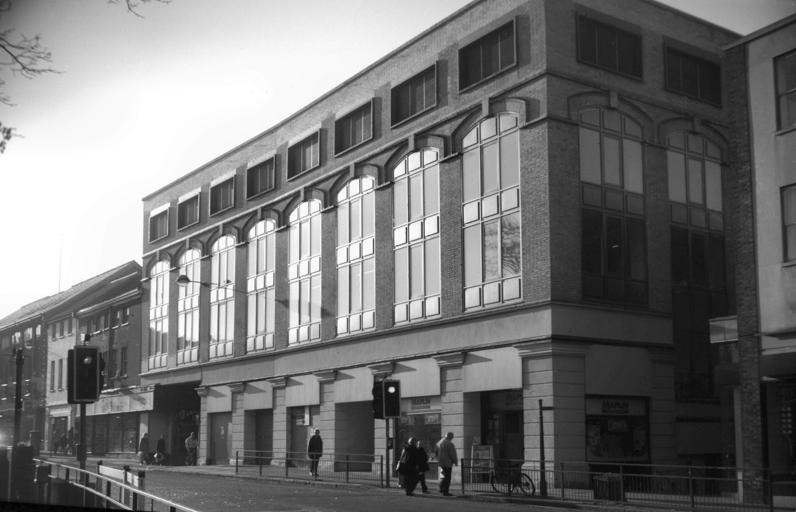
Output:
[471, 445, 493, 473]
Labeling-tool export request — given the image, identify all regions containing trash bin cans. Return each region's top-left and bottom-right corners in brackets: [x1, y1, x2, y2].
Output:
[76, 445, 86, 460]
[594, 472, 623, 499]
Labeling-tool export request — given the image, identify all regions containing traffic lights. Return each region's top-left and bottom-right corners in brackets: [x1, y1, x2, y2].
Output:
[74, 345, 99, 402]
[383, 379, 401, 418]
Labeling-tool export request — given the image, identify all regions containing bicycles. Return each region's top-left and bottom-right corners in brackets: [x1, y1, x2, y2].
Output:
[490, 462, 535, 496]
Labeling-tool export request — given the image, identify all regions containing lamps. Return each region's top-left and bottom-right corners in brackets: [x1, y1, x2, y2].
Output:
[175, 275, 205, 286]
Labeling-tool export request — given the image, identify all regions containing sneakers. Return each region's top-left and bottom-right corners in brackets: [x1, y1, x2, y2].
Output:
[423, 491, 431, 494]
[444, 493, 452, 496]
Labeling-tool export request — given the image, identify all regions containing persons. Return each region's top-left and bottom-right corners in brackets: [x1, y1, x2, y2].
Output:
[307, 428, 325, 478]
[51, 427, 201, 468]
[434, 431, 459, 497]
[414, 439, 431, 494]
[395, 435, 422, 496]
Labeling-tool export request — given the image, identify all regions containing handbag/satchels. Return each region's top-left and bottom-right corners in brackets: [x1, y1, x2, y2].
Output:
[396, 461, 407, 472]
